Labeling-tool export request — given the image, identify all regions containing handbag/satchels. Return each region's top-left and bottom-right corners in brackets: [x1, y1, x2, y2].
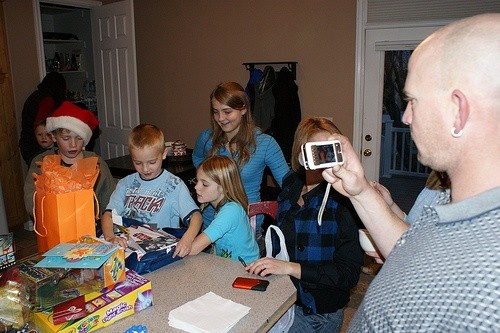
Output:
[33, 188, 100, 253]
[264, 224, 294, 333]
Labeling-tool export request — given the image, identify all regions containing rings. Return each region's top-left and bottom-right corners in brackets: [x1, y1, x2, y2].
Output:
[262, 263, 266, 268]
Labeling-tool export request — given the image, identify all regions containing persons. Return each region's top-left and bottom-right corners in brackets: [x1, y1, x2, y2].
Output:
[101, 123, 203, 258]
[365, 170, 451, 264]
[245, 117, 364, 333]
[19, 72, 116, 234]
[192, 82, 289, 256]
[322, 15, 500, 333]
[189, 156, 260, 265]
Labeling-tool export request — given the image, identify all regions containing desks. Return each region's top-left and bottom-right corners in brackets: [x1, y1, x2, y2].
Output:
[105, 146, 196, 185]
[94, 247, 298, 332]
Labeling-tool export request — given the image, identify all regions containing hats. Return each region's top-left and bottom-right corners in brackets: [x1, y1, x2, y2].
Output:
[45, 100, 100, 150]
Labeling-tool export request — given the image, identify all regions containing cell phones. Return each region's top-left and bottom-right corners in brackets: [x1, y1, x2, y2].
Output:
[232, 277, 269, 291]
[238, 256, 271, 276]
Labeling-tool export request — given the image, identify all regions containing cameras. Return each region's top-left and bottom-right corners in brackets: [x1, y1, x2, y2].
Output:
[298, 140, 344, 170]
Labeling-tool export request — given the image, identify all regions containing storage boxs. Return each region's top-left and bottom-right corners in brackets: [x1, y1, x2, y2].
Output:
[0, 224, 182, 333]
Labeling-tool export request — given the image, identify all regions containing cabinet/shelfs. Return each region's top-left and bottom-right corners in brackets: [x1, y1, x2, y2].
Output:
[43, 39, 92, 109]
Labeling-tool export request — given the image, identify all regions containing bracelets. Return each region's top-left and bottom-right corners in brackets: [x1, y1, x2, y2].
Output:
[390, 201, 394, 207]
